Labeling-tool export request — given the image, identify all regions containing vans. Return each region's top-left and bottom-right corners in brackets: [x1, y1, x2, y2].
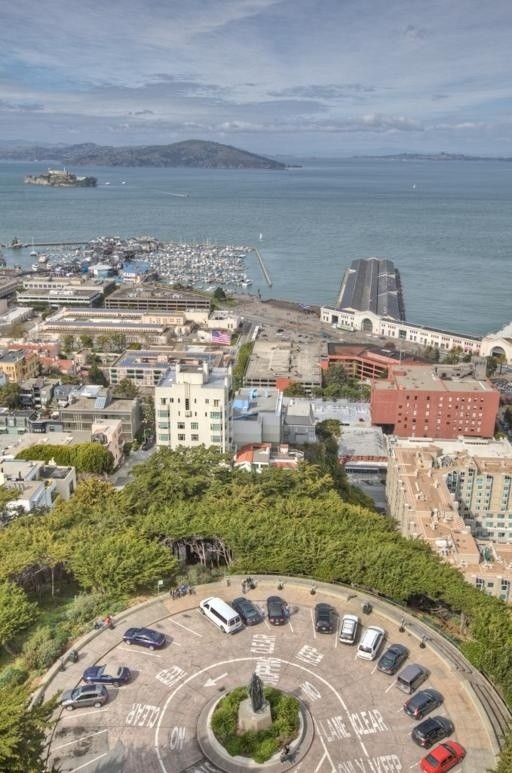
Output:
[199, 594, 242, 634]
[356, 624, 385, 660]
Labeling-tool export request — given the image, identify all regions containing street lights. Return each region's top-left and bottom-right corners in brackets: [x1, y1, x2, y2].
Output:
[43, 478, 53, 513]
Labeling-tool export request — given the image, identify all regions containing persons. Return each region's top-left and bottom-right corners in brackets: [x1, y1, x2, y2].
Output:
[105, 616, 113, 625]
[169, 583, 192, 601]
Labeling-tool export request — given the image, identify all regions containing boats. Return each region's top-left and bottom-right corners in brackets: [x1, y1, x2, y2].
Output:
[22, 167, 100, 189]
[135, 238, 256, 295]
[18, 246, 82, 277]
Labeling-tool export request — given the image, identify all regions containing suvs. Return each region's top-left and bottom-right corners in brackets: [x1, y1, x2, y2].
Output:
[395, 662, 431, 694]
[60, 683, 108, 710]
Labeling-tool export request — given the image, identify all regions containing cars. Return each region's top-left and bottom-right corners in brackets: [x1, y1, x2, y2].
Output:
[402, 687, 444, 719]
[411, 713, 455, 747]
[231, 597, 261, 626]
[314, 601, 334, 633]
[80, 664, 133, 686]
[120, 625, 168, 649]
[338, 612, 359, 644]
[376, 643, 409, 673]
[266, 591, 285, 627]
[419, 739, 466, 772]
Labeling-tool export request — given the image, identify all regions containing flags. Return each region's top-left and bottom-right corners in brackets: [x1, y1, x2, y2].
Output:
[210, 330, 231, 346]
